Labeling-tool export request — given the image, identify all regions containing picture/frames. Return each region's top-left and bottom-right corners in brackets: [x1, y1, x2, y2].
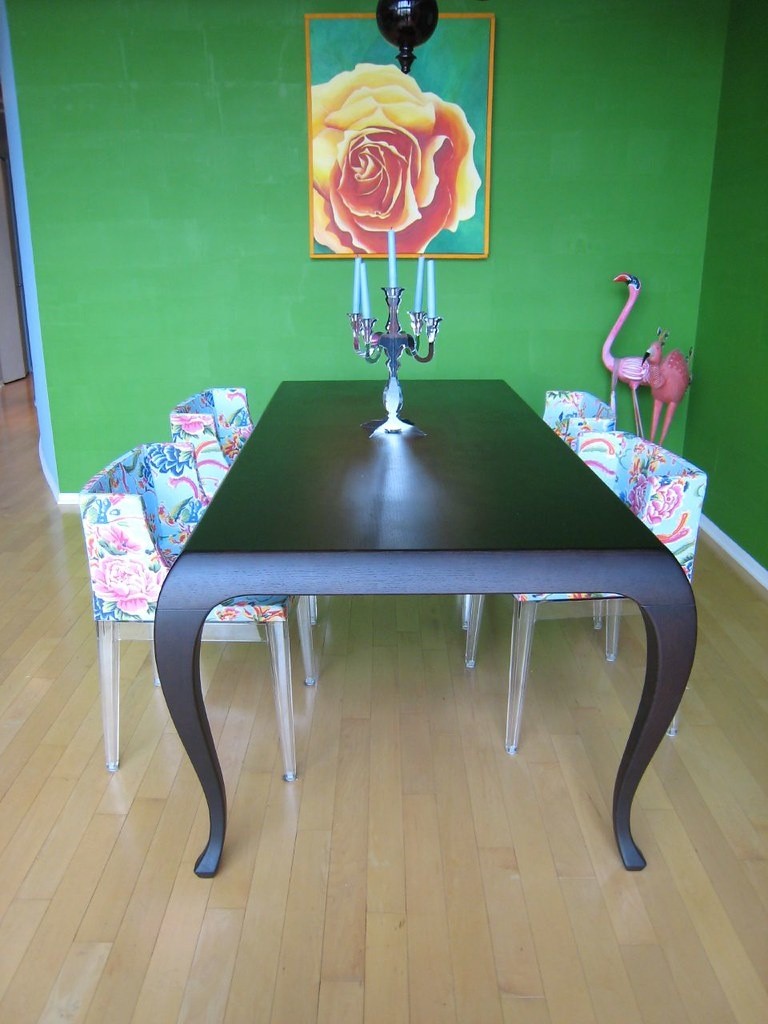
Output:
[303, 12, 495, 261]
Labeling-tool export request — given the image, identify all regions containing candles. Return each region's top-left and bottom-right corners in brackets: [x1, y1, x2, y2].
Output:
[352, 253, 371, 318]
[387, 227, 399, 288]
[413, 254, 436, 317]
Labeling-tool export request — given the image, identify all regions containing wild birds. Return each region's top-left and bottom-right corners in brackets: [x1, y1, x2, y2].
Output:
[602, 273, 650, 438]
[641, 342, 690, 447]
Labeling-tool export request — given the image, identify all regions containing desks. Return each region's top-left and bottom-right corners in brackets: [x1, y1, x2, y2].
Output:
[151, 380, 701, 880]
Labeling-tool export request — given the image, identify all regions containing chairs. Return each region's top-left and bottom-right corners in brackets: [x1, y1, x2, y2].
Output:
[463, 389, 707, 755]
[80, 387, 317, 785]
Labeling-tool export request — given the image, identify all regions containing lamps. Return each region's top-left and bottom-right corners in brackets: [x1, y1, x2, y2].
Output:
[375, 0, 440, 76]
